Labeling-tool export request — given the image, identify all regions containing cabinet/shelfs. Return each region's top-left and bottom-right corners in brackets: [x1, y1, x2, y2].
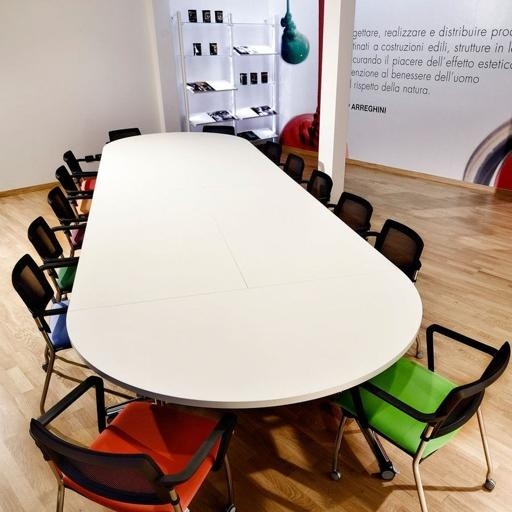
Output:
[177, 9, 279, 146]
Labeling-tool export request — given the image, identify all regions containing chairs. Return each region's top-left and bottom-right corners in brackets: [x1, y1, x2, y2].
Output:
[27, 216, 81, 303]
[326, 322, 511, 511]
[53, 164, 93, 222]
[263, 141, 285, 167]
[201, 125, 238, 136]
[61, 148, 100, 191]
[26, 374, 241, 512]
[47, 184, 88, 258]
[362, 216, 425, 285]
[328, 191, 374, 241]
[299, 169, 335, 210]
[106, 126, 142, 142]
[280, 151, 307, 184]
[12, 253, 71, 420]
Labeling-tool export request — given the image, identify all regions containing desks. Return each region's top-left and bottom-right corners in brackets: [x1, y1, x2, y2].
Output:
[66, 132, 425, 481]
